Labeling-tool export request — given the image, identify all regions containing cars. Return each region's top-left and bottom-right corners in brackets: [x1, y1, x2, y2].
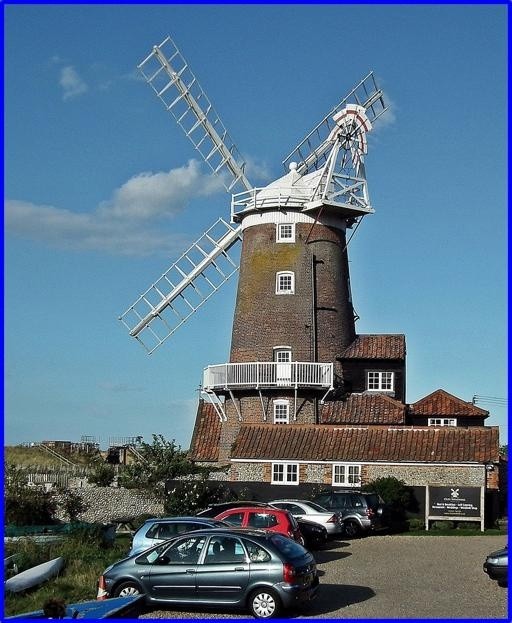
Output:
[97, 484, 391, 618]
[484, 539, 509, 589]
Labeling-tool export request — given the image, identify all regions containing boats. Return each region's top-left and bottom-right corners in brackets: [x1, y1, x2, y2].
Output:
[3, 526, 76, 550]
[5, 556, 64, 595]
